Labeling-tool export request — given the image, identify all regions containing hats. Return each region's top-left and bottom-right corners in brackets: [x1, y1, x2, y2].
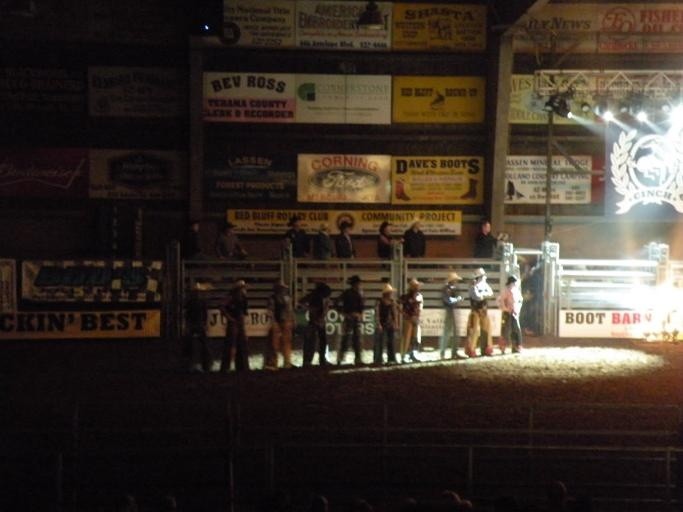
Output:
[224, 221, 237, 229]
[288, 217, 419, 232]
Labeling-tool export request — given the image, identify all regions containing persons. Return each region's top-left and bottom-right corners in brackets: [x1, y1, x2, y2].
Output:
[214, 222, 249, 262]
[400, 217, 426, 259]
[335, 220, 358, 261]
[520, 262, 543, 338]
[437, 271, 464, 361]
[394, 275, 425, 366]
[313, 222, 333, 261]
[294, 281, 333, 367]
[183, 282, 214, 374]
[1, 479, 591, 510]
[496, 272, 521, 355]
[464, 267, 496, 358]
[372, 283, 399, 368]
[217, 279, 251, 374]
[286, 216, 312, 259]
[260, 277, 299, 372]
[474, 221, 496, 263]
[334, 275, 370, 368]
[376, 220, 398, 259]
